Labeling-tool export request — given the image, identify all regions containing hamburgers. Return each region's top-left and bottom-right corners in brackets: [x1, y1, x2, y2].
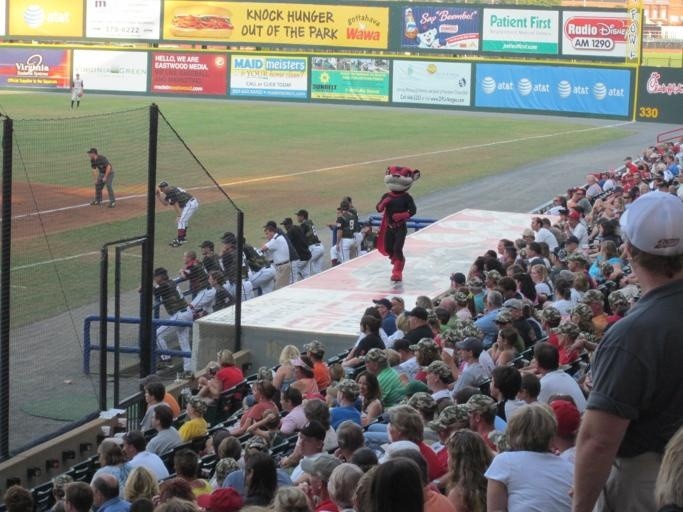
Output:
[169, 5, 234, 40]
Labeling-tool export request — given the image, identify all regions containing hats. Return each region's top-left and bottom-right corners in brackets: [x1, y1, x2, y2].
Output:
[159, 182, 168, 187]
[618, 192, 682, 257]
[54, 342, 359, 511]
[197, 210, 307, 248]
[153, 268, 165, 277]
[341, 201, 349, 210]
[519, 207, 585, 244]
[87, 148, 96, 152]
[366, 249, 629, 481]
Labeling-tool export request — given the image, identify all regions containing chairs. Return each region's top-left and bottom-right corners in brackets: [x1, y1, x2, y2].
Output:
[0, 330, 592, 512]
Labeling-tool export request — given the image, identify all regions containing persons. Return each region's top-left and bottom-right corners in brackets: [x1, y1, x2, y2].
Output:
[71, 74, 83, 109]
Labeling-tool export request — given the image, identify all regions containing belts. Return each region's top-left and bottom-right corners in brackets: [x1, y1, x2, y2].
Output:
[275, 262, 288, 266]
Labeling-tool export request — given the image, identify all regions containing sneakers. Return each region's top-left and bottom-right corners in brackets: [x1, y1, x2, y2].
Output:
[109, 202, 115, 208]
[173, 237, 186, 243]
[168, 241, 183, 246]
[90, 200, 99, 205]
